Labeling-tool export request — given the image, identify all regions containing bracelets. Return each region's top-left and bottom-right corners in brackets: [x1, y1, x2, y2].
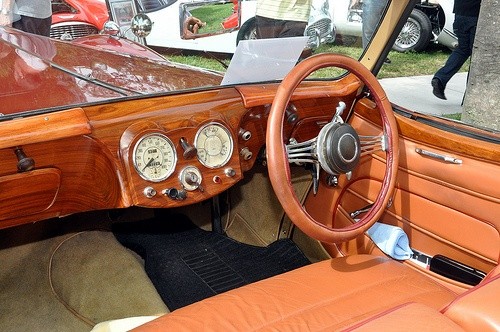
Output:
[2, 10, 11, 15]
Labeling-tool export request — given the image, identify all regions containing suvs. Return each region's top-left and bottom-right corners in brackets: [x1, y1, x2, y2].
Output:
[313, 0, 466, 54]
[112, 1, 336, 56]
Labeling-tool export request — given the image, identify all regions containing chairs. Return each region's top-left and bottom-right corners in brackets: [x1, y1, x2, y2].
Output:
[125, 253, 500, 332]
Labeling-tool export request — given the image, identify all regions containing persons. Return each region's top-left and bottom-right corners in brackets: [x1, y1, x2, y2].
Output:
[0, 0, 51, 37]
[362, 0, 392, 65]
[430, 0, 482, 106]
[255, 0, 311, 39]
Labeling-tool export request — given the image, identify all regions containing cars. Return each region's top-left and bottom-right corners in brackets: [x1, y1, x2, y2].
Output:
[0, 1, 120, 40]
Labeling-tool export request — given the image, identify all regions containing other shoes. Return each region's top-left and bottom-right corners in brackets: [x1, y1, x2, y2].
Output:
[431, 78, 446, 100]
[385, 57, 392, 64]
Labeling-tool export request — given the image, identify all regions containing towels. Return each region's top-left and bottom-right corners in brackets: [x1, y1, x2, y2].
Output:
[354, 218, 415, 262]
